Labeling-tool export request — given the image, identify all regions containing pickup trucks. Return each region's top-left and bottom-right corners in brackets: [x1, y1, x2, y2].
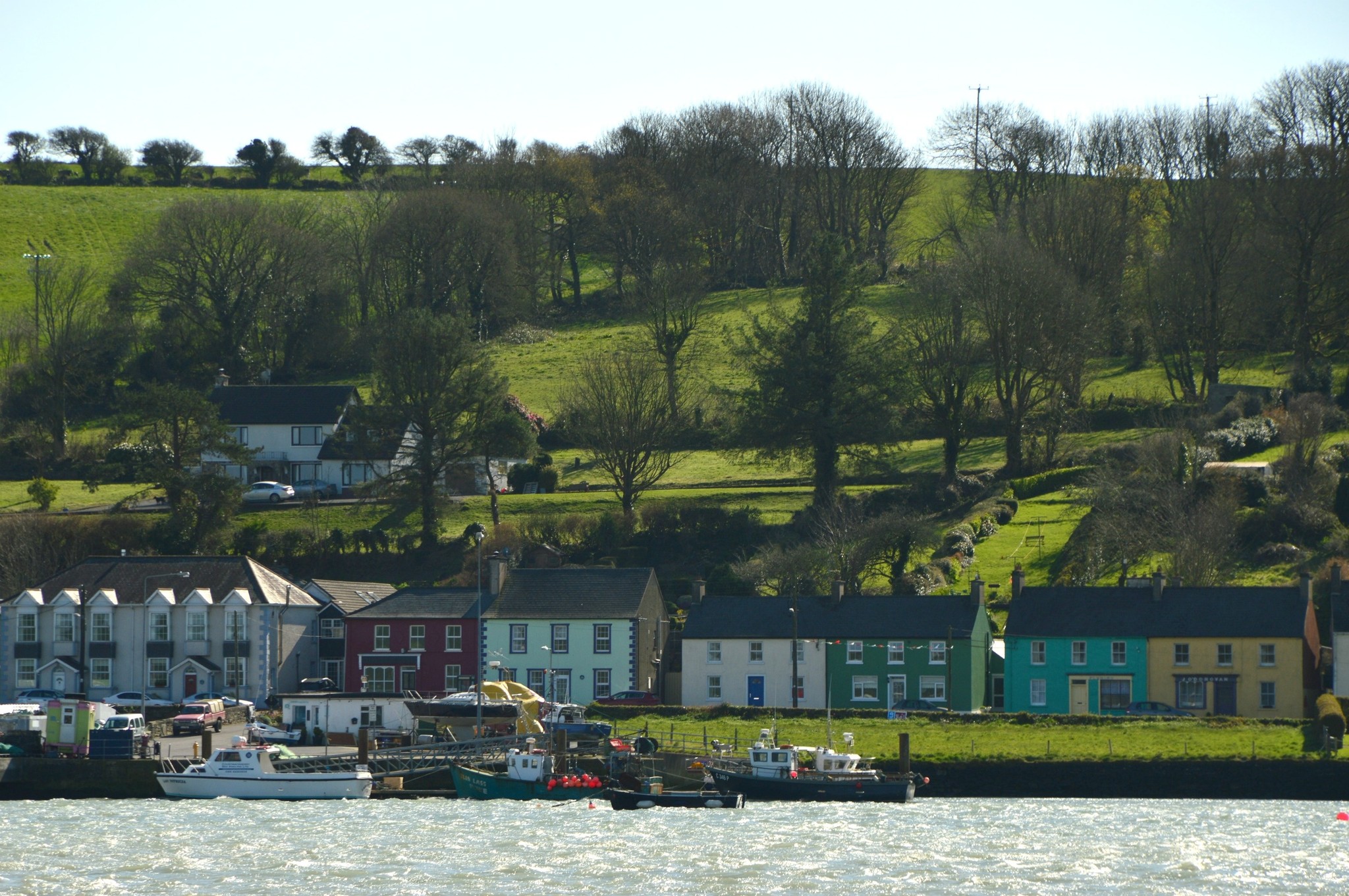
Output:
[172, 699, 225, 737]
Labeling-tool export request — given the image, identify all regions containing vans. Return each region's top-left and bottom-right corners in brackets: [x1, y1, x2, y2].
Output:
[101, 714, 145, 742]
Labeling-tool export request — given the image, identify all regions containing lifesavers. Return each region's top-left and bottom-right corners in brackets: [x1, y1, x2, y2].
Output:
[780, 744, 793, 749]
[534, 749, 547, 753]
[256, 745, 270, 750]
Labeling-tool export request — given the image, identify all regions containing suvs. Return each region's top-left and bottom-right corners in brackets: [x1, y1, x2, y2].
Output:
[298, 677, 341, 692]
[595, 690, 662, 706]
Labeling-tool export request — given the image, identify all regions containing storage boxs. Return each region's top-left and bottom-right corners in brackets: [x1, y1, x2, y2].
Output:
[383, 777, 403, 789]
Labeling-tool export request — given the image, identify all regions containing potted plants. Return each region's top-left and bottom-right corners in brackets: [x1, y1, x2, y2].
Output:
[313, 727, 323, 746]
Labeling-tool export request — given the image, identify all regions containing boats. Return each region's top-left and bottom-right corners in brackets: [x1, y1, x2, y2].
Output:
[398, 665, 932, 811]
[251, 717, 302, 744]
[153, 734, 373, 800]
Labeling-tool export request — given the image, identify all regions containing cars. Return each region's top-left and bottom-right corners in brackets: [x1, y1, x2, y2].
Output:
[182, 692, 253, 709]
[892, 699, 953, 713]
[292, 479, 337, 500]
[102, 691, 175, 706]
[15, 688, 64, 703]
[239, 482, 295, 503]
[1126, 700, 1197, 718]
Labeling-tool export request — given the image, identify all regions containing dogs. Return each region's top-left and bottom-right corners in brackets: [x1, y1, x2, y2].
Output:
[153, 497, 166, 505]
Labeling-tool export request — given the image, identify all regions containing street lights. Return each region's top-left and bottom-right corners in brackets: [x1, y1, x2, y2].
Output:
[141, 570, 190, 720]
[788, 608, 799, 708]
[474, 531, 483, 756]
[21, 252, 51, 357]
[540, 645, 553, 756]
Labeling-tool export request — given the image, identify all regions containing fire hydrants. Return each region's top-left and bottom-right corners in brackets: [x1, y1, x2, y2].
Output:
[193, 741, 200, 759]
